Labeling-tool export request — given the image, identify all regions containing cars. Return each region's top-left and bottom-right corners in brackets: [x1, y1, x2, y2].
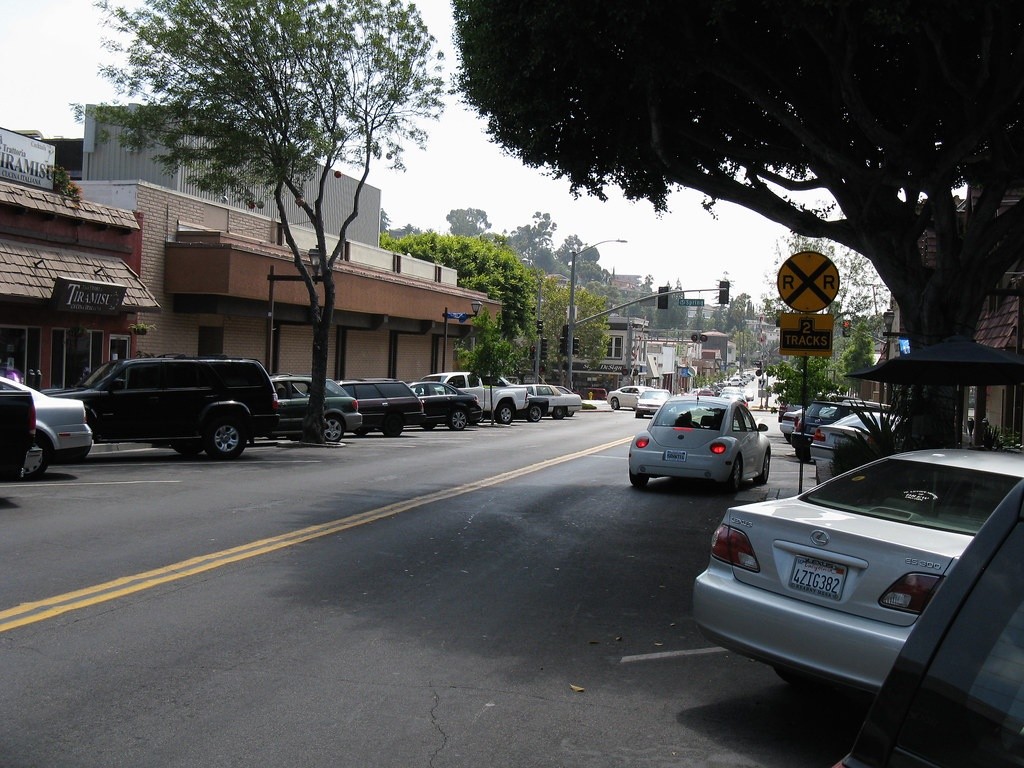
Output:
[689, 368, 755, 406]
[628, 395, 773, 496]
[0, 375, 95, 481]
[516, 385, 583, 420]
[607, 386, 672, 419]
[407, 381, 483, 431]
[686, 447, 1024, 768]
[776, 390, 905, 466]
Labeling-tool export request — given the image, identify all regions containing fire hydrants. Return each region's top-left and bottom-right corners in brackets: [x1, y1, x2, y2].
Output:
[587, 391, 593, 401]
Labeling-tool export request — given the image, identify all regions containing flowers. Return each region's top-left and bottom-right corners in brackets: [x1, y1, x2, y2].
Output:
[48, 163, 80, 199]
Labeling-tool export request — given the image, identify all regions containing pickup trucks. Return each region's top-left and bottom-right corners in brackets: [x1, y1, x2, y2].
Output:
[412, 372, 530, 425]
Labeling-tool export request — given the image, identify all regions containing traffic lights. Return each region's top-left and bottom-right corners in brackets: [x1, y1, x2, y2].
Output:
[842, 319, 851, 338]
[691, 335, 707, 342]
[528, 307, 580, 372]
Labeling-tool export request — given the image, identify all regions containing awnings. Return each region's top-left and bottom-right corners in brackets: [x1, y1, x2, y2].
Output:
[0, 239, 161, 312]
[0, 181, 140, 232]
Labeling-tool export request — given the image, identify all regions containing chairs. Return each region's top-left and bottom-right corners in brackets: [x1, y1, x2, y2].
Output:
[277, 388, 287, 399]
[700, 415, 715, 429]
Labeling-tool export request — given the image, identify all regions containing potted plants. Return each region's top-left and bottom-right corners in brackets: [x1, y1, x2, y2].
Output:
[129, 322, 159, 336]
[67, 324, 88, 337]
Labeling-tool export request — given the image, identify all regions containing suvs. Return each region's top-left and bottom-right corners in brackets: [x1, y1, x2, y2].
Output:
[40, 352, 281, 460]
[270, 374, 427, 439]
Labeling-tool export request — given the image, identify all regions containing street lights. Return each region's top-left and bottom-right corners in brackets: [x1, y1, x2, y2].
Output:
[522, 258, 542, 386]
[566, 239, 629, 392]
[441, 300, 484, 372]
[266, 248, 326, 375]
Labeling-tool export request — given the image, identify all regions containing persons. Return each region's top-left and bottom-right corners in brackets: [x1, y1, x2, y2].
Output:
[967, 418, 975, 436]
[672, 406, 694, 427]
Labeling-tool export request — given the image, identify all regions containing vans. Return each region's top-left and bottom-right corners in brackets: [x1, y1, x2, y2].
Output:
[581, 388, 607, 401]
